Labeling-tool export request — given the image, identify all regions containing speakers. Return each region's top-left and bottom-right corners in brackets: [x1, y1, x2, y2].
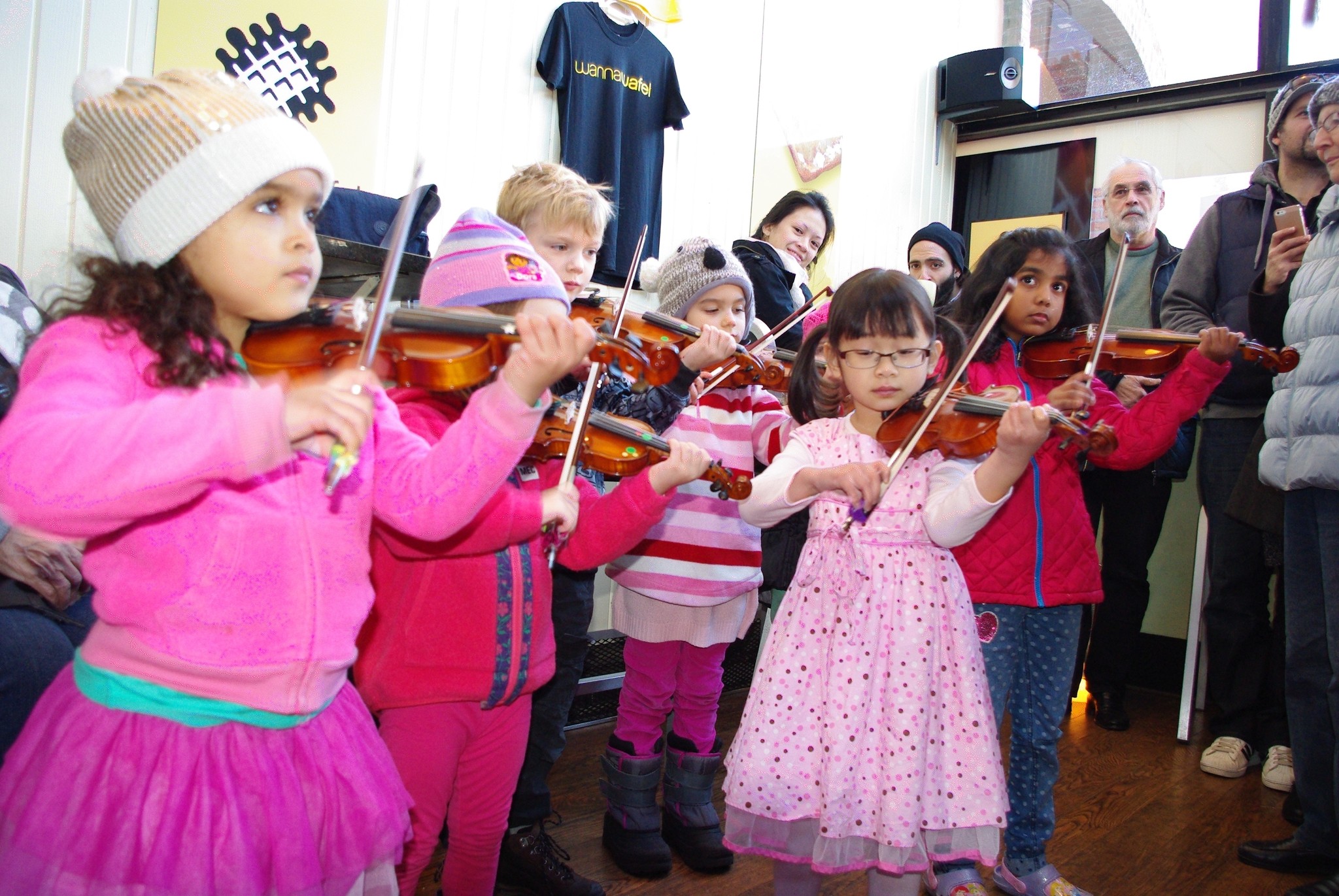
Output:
[935, 44, 1042, 120]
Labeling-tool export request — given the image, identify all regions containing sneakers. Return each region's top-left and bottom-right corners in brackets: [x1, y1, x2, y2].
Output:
[1200, 736, 1261, 778]
[1262, 745, 1295, 793]
[493, 810, 606, 896]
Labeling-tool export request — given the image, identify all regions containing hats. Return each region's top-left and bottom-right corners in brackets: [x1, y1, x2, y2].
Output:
[419, 204, 571, 318]
[1267, 73, 1338, 159]
[801, 301, 832, 352]
[62, 65, 333, 273]
[1307, 74, 1339, 134]
[638, 235, 756, 341]
[908, 222, 966, 273]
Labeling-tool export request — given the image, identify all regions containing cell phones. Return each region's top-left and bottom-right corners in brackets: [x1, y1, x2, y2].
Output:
[1274, 204, 1307, 263]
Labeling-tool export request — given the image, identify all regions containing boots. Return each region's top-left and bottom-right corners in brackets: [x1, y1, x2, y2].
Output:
[662, 753, 734, 872]
[598, 748, 673, 878]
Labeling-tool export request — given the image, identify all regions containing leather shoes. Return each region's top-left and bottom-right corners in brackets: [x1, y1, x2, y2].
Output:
[1237, 833, 1339, 876]
[1284, 877, 1339, 896]
[1086, 693, 1129, 730]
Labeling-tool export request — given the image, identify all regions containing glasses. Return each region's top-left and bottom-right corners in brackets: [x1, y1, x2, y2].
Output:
[1104, 187, 1162, 201]
[836, 344, 936, 369]
[1271, 73, 1338, 114]
[1308, 111, 1339, 146]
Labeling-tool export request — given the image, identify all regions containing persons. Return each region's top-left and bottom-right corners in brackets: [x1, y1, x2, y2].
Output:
[720, 193, 836, 727]
[1234, 74, 1338, 896]
[0, 62, 599, 896]
[905, 222, 972, 316]
[918, 225, 1247, 896]
[723, 268, 1061, 895]
[494, 163, 737, 895]
[593, 237, 851, 880]
[1158, 69, 1334, 793]
[338, 201, 712, 896]
[1049, 162, 1200, 730]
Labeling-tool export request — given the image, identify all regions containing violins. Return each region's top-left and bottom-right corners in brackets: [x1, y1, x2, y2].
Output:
[699, 344, 829, 392]
[240, 293, 680, 392]
[570, 290, 786, 385]
[521, 394, 753, 501]
[1020, 323, 1300, 379]
[876, 380, 1118, 470]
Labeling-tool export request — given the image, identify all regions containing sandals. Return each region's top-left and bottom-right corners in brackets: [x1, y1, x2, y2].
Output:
[992, 856, 1094, 896]
[922, 862, 988, 896]
